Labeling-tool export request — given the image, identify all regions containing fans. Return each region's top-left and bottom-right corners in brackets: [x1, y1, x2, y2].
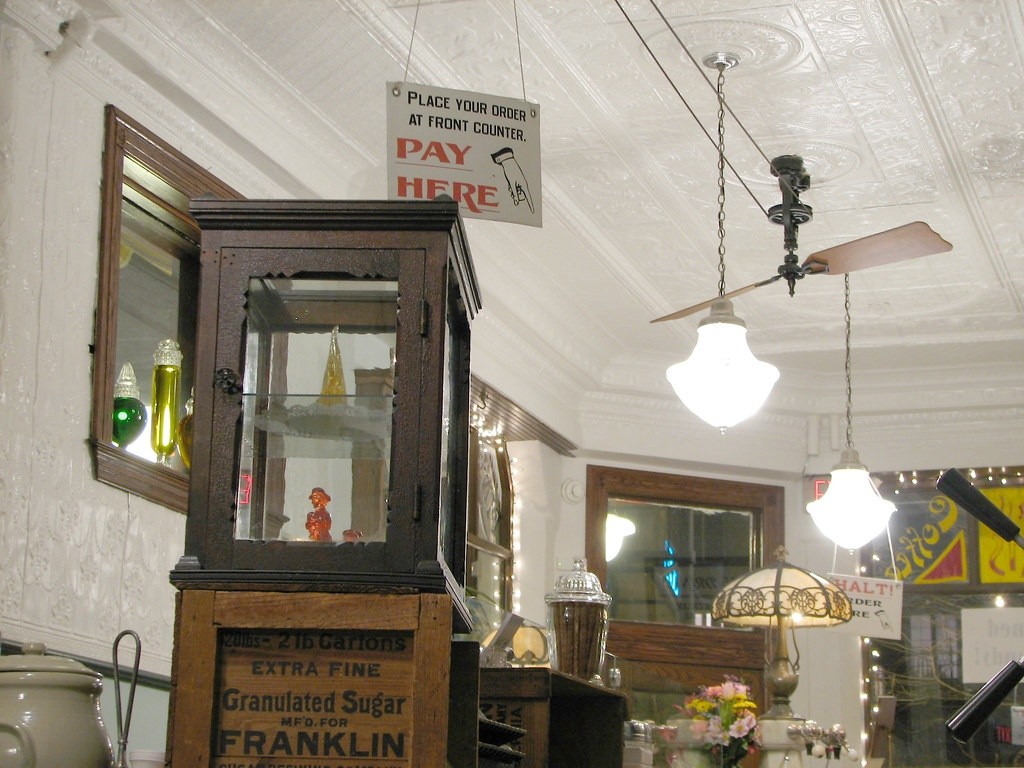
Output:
[648, 155, 952, 323]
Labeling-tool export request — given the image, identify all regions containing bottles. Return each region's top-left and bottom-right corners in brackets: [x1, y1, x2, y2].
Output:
[545, 558, 612, 687]
[113, 338, 196, 477]
[319, 325, 349, 410]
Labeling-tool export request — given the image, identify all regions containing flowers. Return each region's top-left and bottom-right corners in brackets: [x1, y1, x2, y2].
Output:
[673, 674, 762, 768]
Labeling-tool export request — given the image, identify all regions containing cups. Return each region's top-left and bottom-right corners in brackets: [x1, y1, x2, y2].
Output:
[129, 750, 165, 768]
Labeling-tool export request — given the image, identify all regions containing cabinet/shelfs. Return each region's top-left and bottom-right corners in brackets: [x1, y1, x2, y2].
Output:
[163, 193, 632, 768]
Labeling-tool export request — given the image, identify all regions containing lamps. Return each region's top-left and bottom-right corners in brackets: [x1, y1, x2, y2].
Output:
[805, 274, 896, 547]
[665, 54, 781, 430]
[712, 545, 852, 719]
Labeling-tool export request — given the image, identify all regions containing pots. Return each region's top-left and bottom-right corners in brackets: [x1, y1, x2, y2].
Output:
[0, 641, 118, 768]
